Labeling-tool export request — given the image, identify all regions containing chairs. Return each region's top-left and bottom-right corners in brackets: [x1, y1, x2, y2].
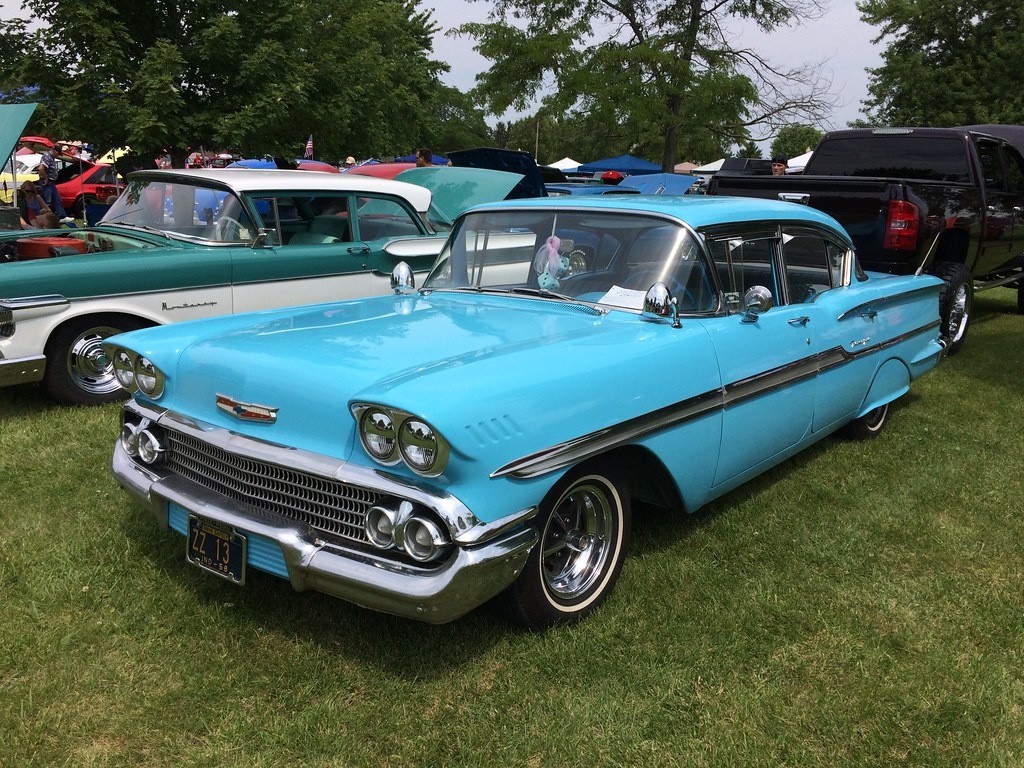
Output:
[83, 204, 114, 227]
[284, 230, 343, 243]
[307, 214, 363, 242]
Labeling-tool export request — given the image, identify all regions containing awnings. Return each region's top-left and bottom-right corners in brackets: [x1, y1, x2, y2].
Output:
[783, 151, 814, 174]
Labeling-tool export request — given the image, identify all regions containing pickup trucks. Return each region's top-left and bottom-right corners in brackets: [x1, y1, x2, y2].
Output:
[705, 124, 1024, 356]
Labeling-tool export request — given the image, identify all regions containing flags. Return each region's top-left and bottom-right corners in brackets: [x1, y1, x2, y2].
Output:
[303, 135, 312, 158]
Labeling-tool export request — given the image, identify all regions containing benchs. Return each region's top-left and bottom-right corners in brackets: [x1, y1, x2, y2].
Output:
[626, 261, 830, 304]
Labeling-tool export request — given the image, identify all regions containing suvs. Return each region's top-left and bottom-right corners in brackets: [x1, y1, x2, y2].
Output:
[188, 153, 209, 166]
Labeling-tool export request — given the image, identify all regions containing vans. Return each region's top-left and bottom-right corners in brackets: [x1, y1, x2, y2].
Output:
[717, 157, 774, 175]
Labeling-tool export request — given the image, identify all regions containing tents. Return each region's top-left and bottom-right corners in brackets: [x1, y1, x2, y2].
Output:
[675, 162, 699, 174]
[542, 157, 593, 173]
[393, 154, 449, 165]
[576, 155, 662, 183]
[689, 158, 726, 185]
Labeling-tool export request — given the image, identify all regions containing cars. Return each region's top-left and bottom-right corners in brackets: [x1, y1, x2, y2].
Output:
[20, 135, 128, 220]
[166, 159, 333, 229]
[0, 102, 537, 405]
[297, 161, 434, 220]
[213, 154, 234, 167]
[99, 194, 951, 629]
[445, 147, 697, 237]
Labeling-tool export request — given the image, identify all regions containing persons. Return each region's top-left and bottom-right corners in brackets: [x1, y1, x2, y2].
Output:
[193, 154, 204, 168]
[12, 180, 59, 231]
[415, 148, 432, 167]
[771, 155, 789, 175]
[162, 160, 170, 169]
[345, 157, 357, 169]
[61, 145, 88, 166]
[38, 145, 75, 223]
